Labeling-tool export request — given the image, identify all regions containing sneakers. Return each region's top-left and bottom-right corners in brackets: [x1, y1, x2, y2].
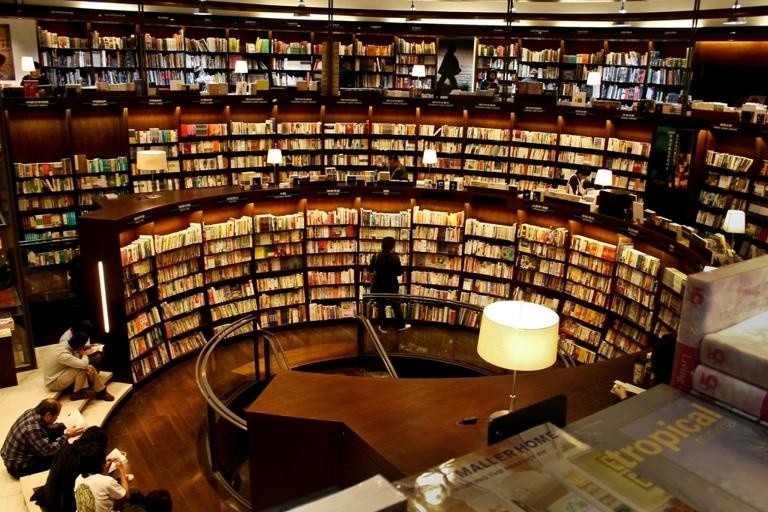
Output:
[379, 326, 387, 333]
[398, 324, 411, 330]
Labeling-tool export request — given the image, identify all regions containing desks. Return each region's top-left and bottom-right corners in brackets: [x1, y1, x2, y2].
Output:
[0, 285, 24, 389]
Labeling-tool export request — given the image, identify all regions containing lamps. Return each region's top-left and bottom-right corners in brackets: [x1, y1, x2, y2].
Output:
[21, 56, 36, 72]
[611, 10, 632, 27]
[294, 3, 310, 17]
[136, 150, 167, 170]
[97, 261, 111, 334]
[476, 300, 560, 421]
[193, 0, 213, 15]
[412, 65, 425, 77]
[405, 7, 422, 22]
[723, 210, 745, 234]
[723, 4, 747, 25]
[234, 61, 248, 74]
[586, 71, 601, 85]
[504, 7, 520, 23]
[423, 149, 438, 164]
[594, 169, 612, 186]
[267, 149, 283, 164]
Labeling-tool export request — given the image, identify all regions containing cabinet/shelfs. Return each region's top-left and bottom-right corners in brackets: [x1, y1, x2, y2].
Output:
[37, 18, 768, 108]
[80, 182, 710, 386]
[6, 108, 768, 368]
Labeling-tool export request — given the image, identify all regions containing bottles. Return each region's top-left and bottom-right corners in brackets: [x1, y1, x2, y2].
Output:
[633, 356, 646, 385]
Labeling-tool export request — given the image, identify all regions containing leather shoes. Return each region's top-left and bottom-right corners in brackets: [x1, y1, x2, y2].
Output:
[71, 391, 91, 400]
[97, 392, 114, 401]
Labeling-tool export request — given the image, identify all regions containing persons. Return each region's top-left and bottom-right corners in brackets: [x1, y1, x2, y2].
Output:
[388, 155, 408, 180]
[58, 314, 103, 374]
[435, 36, 462, 96]
[74, 444, 145, 512]
[144, 489, 172, 512]
[0, 398, 86, 478]
[480, 68, 500, 94]
[43, 333, 115, 402]
[193, 66, 208, 91]
[566, 165, 591, 196]
[31, 426, 107, 512]
[368, 237, 411, 333]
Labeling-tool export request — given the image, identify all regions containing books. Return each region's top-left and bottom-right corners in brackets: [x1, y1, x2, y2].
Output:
[62, 410, 87, 444]
[557, 299, 607, 364]
[358, 207, 412, 267]
[388, 421, 698, 511]
[179, 124, 230, 188]
[646, 51, 686, 103]
[564, 233, 616, 309]
[230, 120, 274, 186]
[395, 38, 436, 90]
[410, 269, 460, 325]
[283, 472, 409, 511]
[475, 43, 519, 93]
[559, 49, 604, 98]
[185, 37, 227, 84]
[38, 26, 91, 86]
[462, 218, 517, 280]
[91, 343, 104, 352]
[255, 272, 307, 328]
[13, 158, 80, 266]
[416, 123, 464, 181]
[510, 130, 556, 192]
[514, 222, 568, 292]
[154, 223, 203, 300]
[203, 215, 253, 284]
[558, 132, 605, 193]
[274, 122, 322, 183]
[673, 252, 768, 424]
[464, 127, 510, 183]
[307, 269, 357, 321]
[696, 149, 753, 231]
[306, 207, 359, 267]
[744, 159, 768, 243]
[563, 384, 768, 510]
[359, 268, 410, 321]
[518, 47, 561, 90]
[120, 235, 155, 314]
[312, 41, 353, 81]
[457, 276, 510, 329]
[611, 245, 659, 332]
[160, 292, 208, 359]
[206, 281, 258, 341]
[106, 447, 128, 474]
[73, 153, 129, 216]
[654, 267, 688, 339]
[512, 285, 559, 312]
[126, 306, 170, 383]
[370, 122, 416, 182]
[144, 29, 185, 86]
[91, 31, 140, 85]
[606, 137, 651, 192]
[355, 39, 395, 89]
[412, 204, 465, 272]
[601, 50, 648, 100]
[323, 122, 370, 183]
[271, 38, 312, 87]
[597, 318, 650, 362]
[128, 128, 182, 193]
[254, 212, 305, 273]
[228, 37, 269, 86]
